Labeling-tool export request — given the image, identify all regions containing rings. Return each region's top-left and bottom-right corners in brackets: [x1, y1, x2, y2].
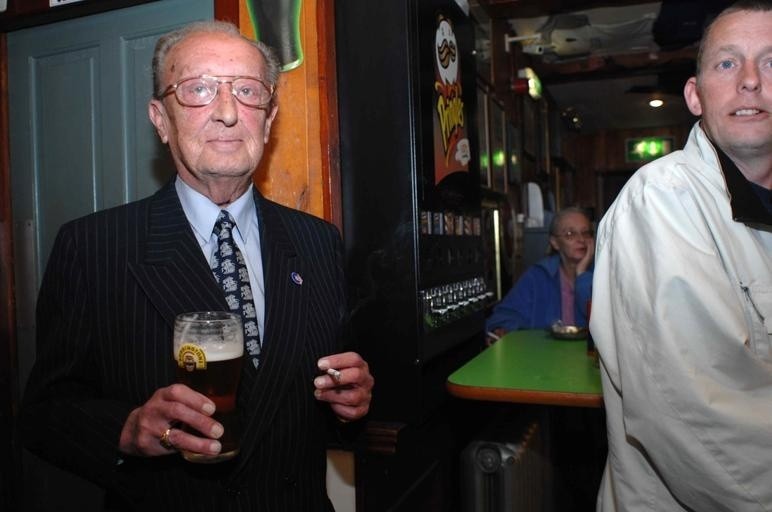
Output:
[160, 426, 173, 448]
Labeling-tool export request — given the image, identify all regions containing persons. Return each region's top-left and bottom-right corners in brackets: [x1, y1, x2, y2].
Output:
[584, 3, 772, 512]
[16, 20, 377, 509]
[484, 203, 609, 508]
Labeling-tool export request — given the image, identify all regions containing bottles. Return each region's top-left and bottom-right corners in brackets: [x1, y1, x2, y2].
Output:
[173, 311, 245, 463]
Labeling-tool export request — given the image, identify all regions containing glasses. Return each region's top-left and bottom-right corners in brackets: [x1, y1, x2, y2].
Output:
[159, 74, 274, 108]
[556, 230, 592, 240]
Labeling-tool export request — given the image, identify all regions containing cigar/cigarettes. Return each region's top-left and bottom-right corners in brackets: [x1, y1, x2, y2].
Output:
[325, 365, 340, 381]
[485, 329, 500, 341]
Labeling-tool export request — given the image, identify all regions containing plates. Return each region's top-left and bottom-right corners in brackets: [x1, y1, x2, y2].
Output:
[547, 322, 585, 341]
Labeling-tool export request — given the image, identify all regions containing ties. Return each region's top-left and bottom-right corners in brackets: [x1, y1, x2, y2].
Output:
[211, 210, 261, 369]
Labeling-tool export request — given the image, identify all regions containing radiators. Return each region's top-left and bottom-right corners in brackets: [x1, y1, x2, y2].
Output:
[460, 410, 554, 511]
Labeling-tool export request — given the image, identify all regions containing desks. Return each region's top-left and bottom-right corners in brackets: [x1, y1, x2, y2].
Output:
[445, 329, 605, 407]
